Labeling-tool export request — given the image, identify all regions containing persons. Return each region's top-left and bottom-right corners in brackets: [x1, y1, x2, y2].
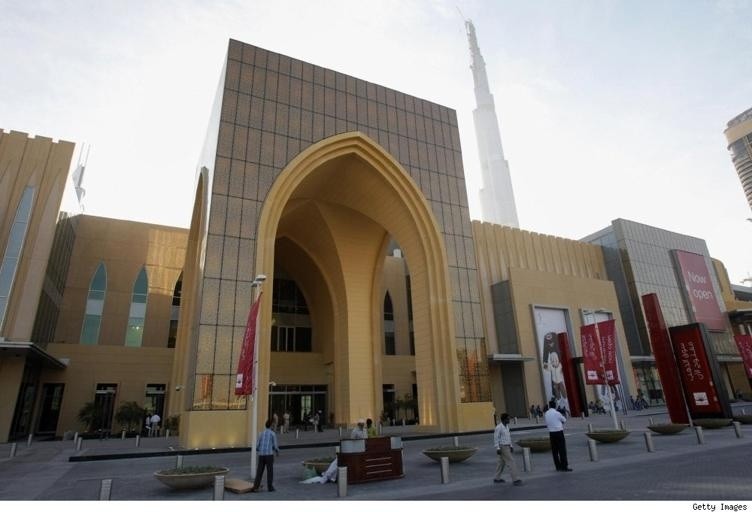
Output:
[146, 414, 151, 436]
[150, 412, 161, 437]
[365, 418, 377, 437]
[351, 419, 368, 440]
[251, 419, 280, 492]
[544, 400, 574, 472]
[542, 332, 569, 399]
[528, 392, 649, 420]
[492, 412, 522, 485]
[268, 406, 324, 432]
[544, 352, 567, 400]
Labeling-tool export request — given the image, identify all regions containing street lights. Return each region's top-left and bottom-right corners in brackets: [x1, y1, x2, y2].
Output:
[583, 307, 620, 429]
[250, 275, 267, 484]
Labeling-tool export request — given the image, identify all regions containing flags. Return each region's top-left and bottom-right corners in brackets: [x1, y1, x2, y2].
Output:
[580, 324, 606, 384]
[234, 293, 262, 396]
[597, 320, 619, 384]
